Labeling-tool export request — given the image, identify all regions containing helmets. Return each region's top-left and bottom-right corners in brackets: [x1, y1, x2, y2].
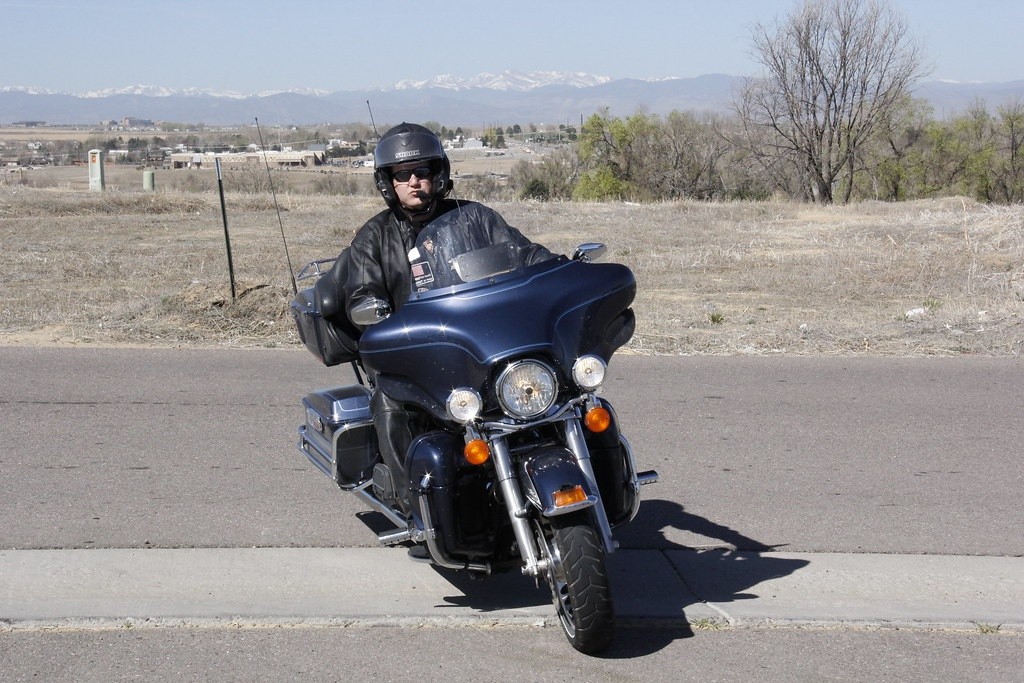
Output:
[372, 121, 453, 224]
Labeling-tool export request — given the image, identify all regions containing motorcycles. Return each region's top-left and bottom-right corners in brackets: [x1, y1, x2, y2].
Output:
[292, 241, 659, 655]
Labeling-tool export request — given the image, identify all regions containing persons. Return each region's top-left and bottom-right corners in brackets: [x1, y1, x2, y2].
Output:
[343, 121, 560, 558]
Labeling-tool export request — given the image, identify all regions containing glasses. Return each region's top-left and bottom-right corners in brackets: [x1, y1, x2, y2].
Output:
[391, 166, 434, 182]
